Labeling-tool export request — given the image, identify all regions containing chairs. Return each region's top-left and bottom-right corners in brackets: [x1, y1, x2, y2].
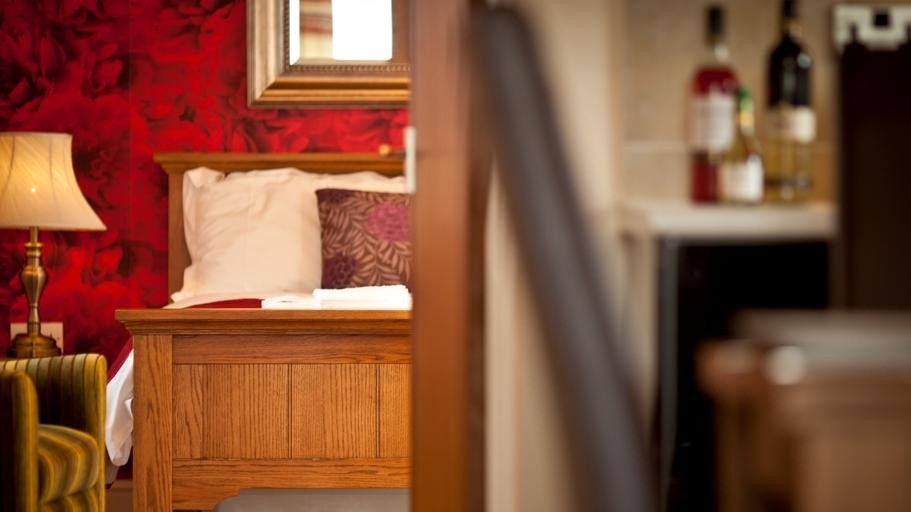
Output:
[0, 353, 107, 512]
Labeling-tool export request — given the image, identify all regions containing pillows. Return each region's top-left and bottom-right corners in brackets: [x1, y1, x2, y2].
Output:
[170, 167, 413, 303]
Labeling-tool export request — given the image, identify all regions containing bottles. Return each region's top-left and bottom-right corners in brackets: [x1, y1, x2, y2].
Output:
[686, 0, 816, 203]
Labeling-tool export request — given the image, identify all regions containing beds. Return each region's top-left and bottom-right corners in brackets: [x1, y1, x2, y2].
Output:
[115, 153, 413, 512]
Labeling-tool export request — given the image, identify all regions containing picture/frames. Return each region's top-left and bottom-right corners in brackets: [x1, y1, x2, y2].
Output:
[246, 0, 410, 109]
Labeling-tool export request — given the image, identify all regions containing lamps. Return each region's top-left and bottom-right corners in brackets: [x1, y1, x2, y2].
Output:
[0, 132, 108, 359]
